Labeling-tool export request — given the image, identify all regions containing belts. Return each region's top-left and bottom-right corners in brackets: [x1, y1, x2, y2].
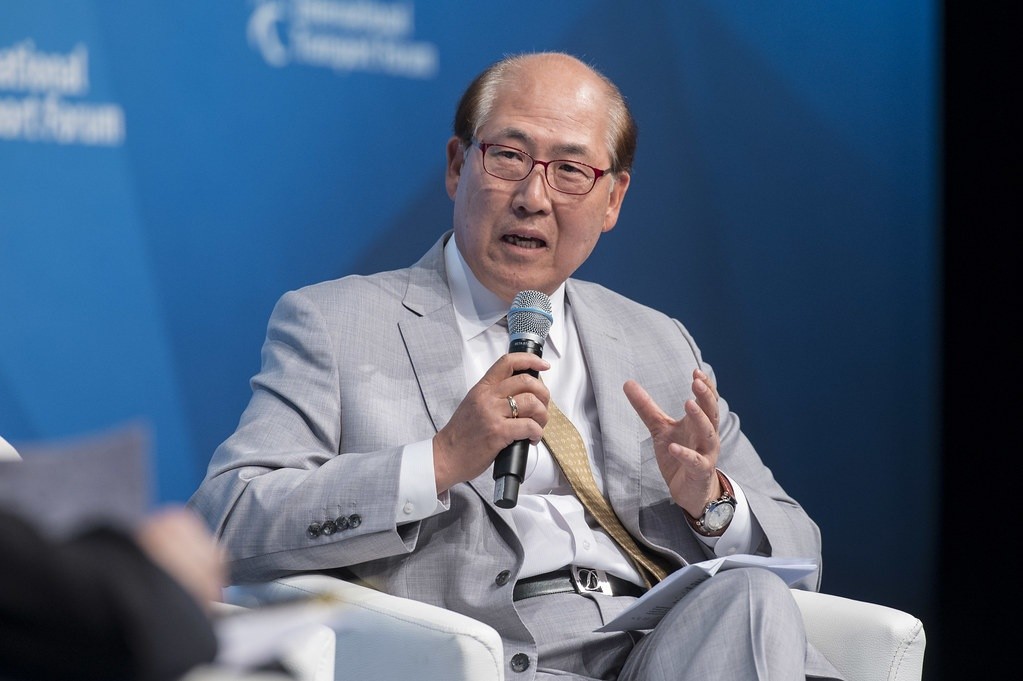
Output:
[513, 568, 651, 601]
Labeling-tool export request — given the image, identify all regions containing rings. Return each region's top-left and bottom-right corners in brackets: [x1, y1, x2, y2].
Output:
[507, 396, 518, 418]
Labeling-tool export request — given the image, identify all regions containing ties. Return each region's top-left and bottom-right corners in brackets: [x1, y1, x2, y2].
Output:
[530, 374, 670, 588]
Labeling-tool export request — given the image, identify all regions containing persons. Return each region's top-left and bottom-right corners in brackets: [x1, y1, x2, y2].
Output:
[187, 52, 845, 681]
[0, 509, 218, 681]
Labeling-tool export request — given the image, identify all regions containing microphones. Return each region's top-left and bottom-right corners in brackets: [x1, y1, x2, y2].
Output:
[493, 290, 554, 510]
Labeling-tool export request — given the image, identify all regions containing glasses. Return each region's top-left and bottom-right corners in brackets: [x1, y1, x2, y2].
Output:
[465, 136, 621, 195]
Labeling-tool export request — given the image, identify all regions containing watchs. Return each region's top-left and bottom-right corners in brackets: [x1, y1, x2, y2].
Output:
[683, 470, 738, 536]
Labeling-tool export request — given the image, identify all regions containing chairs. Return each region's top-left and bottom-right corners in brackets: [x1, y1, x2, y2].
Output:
[211, 574, 926, 681]
